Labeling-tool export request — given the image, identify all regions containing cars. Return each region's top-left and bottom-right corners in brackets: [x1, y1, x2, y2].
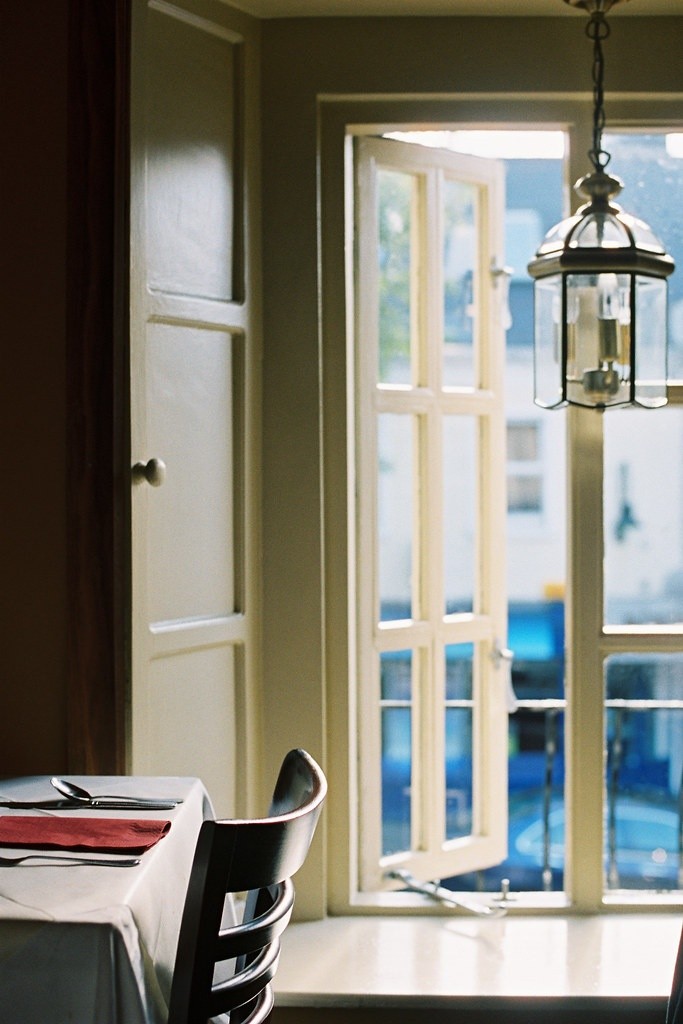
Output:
[423, 792, 683, 890]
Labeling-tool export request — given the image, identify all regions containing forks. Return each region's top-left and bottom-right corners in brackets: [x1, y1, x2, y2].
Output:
[0, 853, 141, 867]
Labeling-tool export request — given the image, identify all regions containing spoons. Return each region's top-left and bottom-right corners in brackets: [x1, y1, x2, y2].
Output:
[50, 777, 184, 805]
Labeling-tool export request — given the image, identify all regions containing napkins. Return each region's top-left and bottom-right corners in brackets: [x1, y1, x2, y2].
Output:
[0, 814, 170, 858]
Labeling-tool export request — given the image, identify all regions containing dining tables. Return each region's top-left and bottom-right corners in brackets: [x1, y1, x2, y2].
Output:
[1, 772, 235, 1024]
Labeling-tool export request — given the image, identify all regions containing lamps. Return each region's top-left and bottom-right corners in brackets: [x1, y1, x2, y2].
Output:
[527, 3, 677, 414]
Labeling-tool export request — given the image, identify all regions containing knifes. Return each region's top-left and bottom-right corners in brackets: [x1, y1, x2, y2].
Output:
[36, 804, 177, 810]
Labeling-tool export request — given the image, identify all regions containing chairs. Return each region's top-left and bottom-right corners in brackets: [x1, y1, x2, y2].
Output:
[165, 746, 330, 1024]
